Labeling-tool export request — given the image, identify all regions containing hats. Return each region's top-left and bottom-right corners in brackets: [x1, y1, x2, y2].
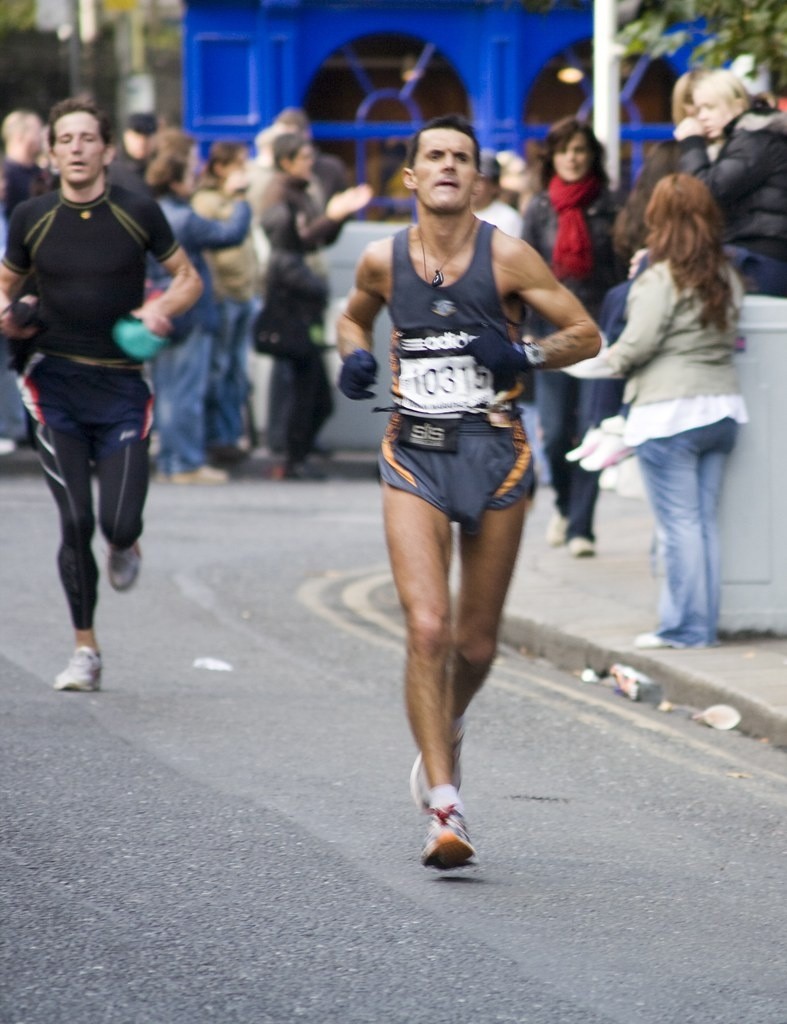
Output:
[131, 114, 160, 134]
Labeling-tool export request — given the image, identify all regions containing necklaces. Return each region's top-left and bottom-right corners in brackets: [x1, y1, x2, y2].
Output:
[419, 224, 471, 286]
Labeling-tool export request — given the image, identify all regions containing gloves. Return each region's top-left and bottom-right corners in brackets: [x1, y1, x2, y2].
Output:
[466, 327, 546, 376]
[339, 350, 377, 401]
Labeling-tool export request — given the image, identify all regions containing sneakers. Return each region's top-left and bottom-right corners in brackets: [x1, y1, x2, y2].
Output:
[408, 719, 465, 812]
[108, 537, 140, 592]
[420, 803, 474, 868]
[55, 645, 102, 692]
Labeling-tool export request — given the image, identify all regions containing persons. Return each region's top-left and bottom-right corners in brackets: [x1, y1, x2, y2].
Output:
[525, 173, 747, 651]
[470, 149, 523, 242]
[0, 97, 204, 693]
[520, 116, 630, 557]
[1, 109, 373, 484]
[337, 114, 601, 870]
[565, 68, 785, 472]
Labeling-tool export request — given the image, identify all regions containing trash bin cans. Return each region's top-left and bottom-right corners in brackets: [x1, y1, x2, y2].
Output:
[714, 294, 787, 637]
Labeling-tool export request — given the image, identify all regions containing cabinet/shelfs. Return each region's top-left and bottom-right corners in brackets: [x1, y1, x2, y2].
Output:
[656, 294, 787, 637]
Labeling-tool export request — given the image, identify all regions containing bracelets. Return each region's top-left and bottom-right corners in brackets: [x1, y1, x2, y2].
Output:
[522, 341, 545, 369]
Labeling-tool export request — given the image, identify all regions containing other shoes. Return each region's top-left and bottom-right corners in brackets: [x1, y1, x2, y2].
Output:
[636, 631, 672, 649]
[563, 354, 625, 378]
[172, 460, 230, 484]
[548, 511, 567, 547]
[567, 534, 596, 557]
[274, 456, 325, 478]
[564, 426, 637, 472]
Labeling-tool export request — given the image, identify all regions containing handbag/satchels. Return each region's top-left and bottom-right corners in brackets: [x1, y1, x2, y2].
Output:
[253, 296, 316, 361]
